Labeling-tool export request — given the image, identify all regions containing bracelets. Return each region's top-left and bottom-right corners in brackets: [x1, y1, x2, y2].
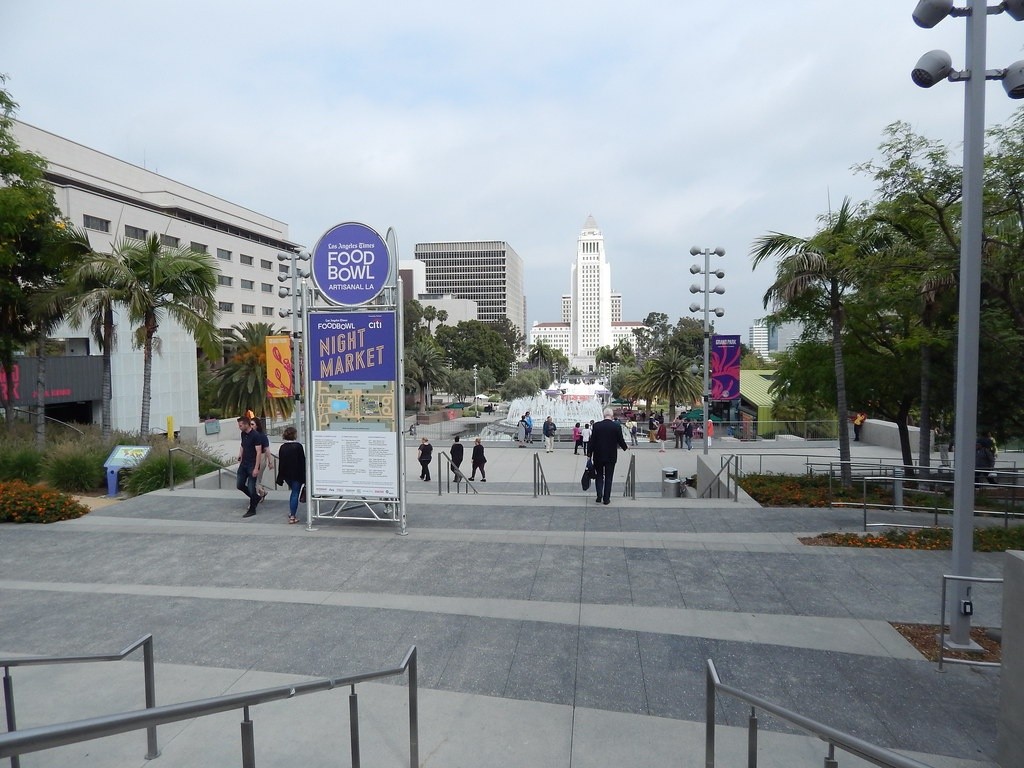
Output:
[269, 461, 272, 464]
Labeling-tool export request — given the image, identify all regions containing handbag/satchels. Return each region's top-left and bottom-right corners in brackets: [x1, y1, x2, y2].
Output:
[300, 486, 306, 503]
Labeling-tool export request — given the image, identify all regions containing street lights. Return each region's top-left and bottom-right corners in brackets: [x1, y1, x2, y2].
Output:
[689, 245, 726, 455]
[277, 249, 313, 445]
[473, 364, 478, 416]
[912, 0, 1024, 651]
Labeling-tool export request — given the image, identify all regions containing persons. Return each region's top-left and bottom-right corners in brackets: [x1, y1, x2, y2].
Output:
[626, 416, 638, 446]
[517, 412, 533, 447]
[236, 407, 263, 517]
[673, 415, 694, 450]
[697, 418, 712, 446]
[418, 437, 433, 481]
[276, 427, 306, 523]
[573, 420, 594, 456]
[649, 412, 666, 451]
[587, 408, 629, 505]
[409, 423, 417, 436]
[543, 416, 557, 453]
[248, 417, 274, 509]
[937, 432, 950, 467]
[975, 430, 999, 488]
[468, 438, 488, 482]
[450, 436, 463, 482]
[488, 402, 496, 415]
[849, 412, 866, 440]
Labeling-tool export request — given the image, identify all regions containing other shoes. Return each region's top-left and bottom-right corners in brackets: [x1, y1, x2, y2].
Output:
[424, 478, 431, 481]
[574, 453, 580, 455]
[242, 510, 255, 518]
[604, 500, 610, 505]
[480, 479, 486, 482]
[420, 476, 424, 479]
[260, 491, 268, 503]
[596, 496, 601, 502]
[468, 478, 474, 481]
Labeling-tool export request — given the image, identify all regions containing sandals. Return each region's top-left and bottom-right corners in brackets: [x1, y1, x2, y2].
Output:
[289, 514, 298, 524]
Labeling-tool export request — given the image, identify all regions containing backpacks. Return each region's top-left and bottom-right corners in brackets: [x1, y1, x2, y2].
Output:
[632, 426, 636, 434]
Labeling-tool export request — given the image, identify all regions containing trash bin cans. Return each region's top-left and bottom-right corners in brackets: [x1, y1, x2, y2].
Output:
[663, 479, 680, 498]
[662, 467, 678, 489]
